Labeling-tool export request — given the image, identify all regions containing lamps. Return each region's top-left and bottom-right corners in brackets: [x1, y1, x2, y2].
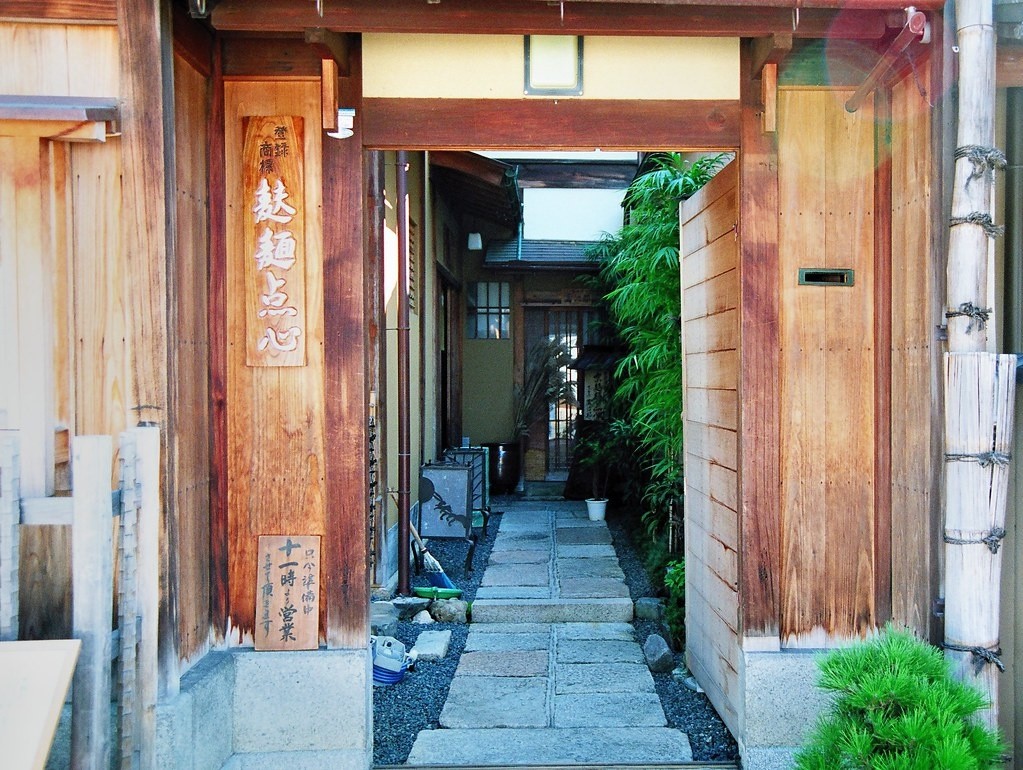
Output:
[468, 212, 482, 251]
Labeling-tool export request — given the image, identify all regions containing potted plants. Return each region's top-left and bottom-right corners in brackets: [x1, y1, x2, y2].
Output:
[476, 333, 582, 494]
[582, 431, 617, 521]
